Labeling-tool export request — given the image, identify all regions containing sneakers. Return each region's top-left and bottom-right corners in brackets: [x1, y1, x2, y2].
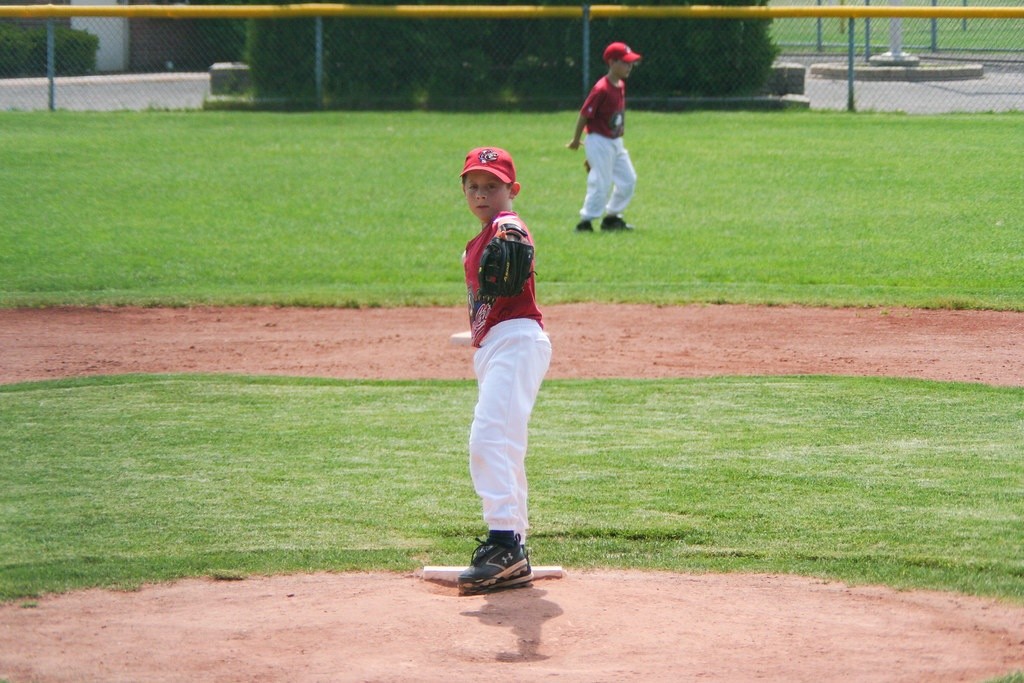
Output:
[456, 533, 528, 587]
[576, 219, 595, 231]
[468, 544, 533, 594]
[601, 215, 634, 231]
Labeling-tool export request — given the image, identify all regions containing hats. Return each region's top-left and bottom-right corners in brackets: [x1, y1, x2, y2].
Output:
[460, 147, 516, 184]
[603, 42, 641, 62]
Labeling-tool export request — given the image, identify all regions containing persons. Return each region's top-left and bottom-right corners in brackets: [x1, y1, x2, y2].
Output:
[457, 148, 552, 596]
[566, 41, 641, 232]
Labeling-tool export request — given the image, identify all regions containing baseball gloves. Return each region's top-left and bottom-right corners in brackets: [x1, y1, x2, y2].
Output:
[475, 222, 539, 309]
